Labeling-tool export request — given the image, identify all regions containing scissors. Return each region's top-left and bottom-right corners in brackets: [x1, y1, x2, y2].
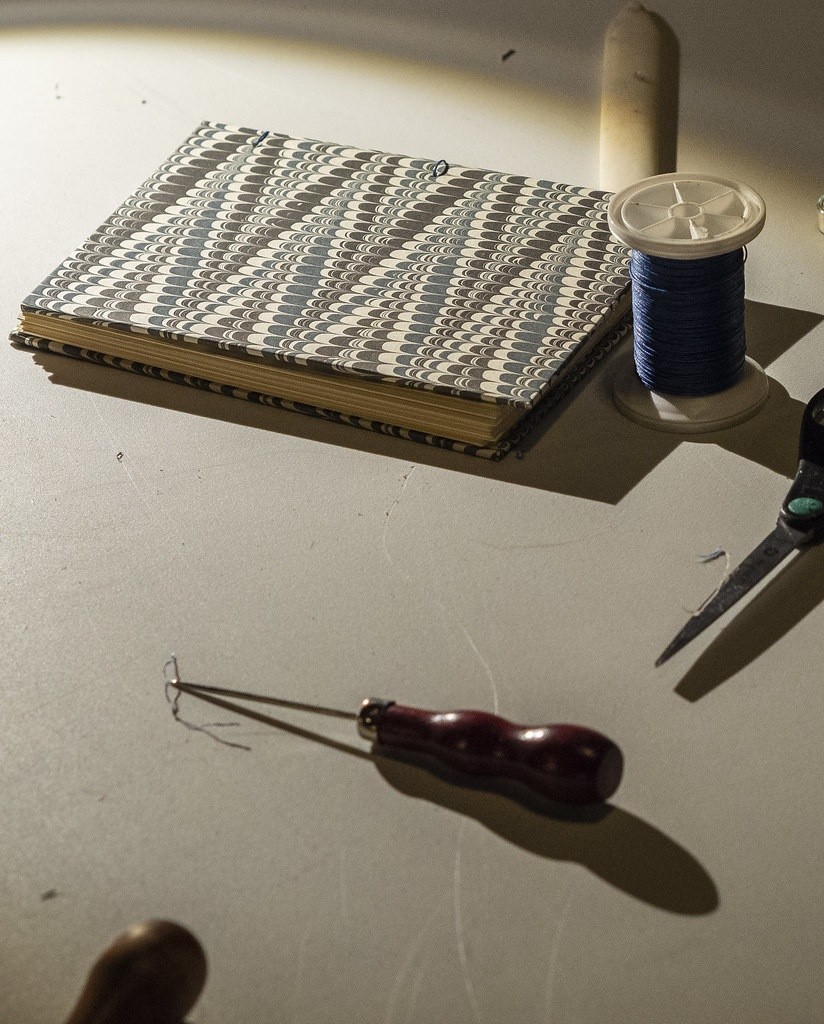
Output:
[652, 386, 824, 672]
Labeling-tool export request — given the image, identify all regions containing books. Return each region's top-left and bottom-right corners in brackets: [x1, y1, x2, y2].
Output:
[7, 115, 636, 461]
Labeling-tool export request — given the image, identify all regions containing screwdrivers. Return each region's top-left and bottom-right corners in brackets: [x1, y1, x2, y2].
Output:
[169, 678, 625, 804]
